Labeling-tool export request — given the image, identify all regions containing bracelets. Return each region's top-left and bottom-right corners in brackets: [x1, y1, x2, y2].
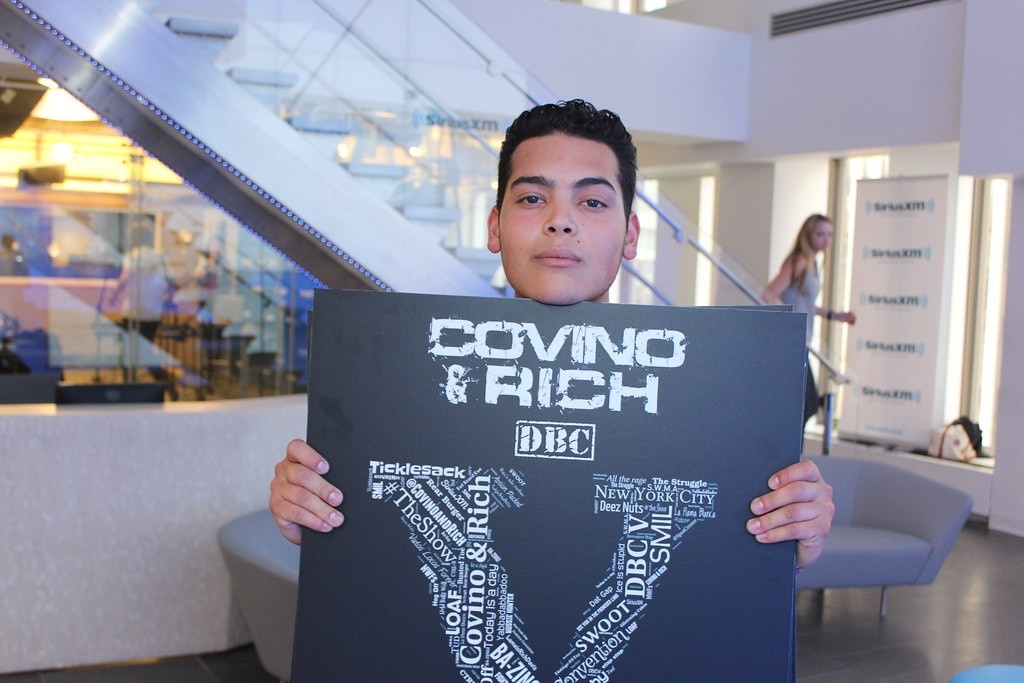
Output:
[827, 311, 833, 320]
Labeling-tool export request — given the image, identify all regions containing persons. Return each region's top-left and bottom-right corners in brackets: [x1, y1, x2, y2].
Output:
[0, 236, 29, 275]
[109, 219, 180, 401]
[758, 211, 858, 455]
[266, 96, 836, 574]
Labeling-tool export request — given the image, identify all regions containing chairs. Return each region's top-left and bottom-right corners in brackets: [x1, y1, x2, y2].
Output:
[194, 324, 278, 398]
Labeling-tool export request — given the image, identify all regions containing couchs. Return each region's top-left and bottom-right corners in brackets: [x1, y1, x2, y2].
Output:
[217, 456, 974, 683]
